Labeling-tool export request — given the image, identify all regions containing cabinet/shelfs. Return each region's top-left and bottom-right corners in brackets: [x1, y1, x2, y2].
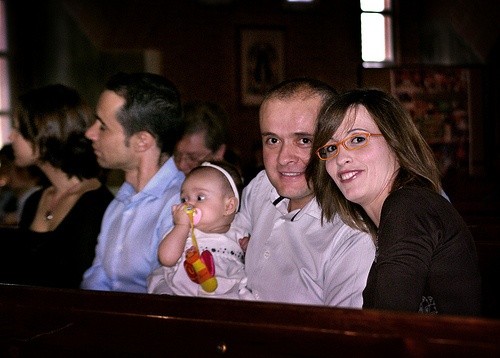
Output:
[390, 63, 483, 174]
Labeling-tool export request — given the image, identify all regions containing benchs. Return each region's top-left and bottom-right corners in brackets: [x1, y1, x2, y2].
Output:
[0, 284, 500, 358]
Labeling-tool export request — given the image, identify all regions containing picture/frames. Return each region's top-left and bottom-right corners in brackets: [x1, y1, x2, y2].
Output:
[233, 23, 288, 109]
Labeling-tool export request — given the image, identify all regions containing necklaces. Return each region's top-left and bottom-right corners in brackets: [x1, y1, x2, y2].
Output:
[45, 208, 56, 220]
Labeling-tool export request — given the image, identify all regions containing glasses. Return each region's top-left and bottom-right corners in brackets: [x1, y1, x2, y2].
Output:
[315, 132, 384, 160]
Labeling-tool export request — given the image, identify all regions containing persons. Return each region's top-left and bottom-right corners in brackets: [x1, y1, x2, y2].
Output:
[304, 89, 484, 318]
[167, 96, 245, 181]
[156, 160, 251, 301]
[150, 79, 375, 310]
[7, 80, 115, 290]
[82, 71, 187, 293]
[0, 142, 46, 231]
